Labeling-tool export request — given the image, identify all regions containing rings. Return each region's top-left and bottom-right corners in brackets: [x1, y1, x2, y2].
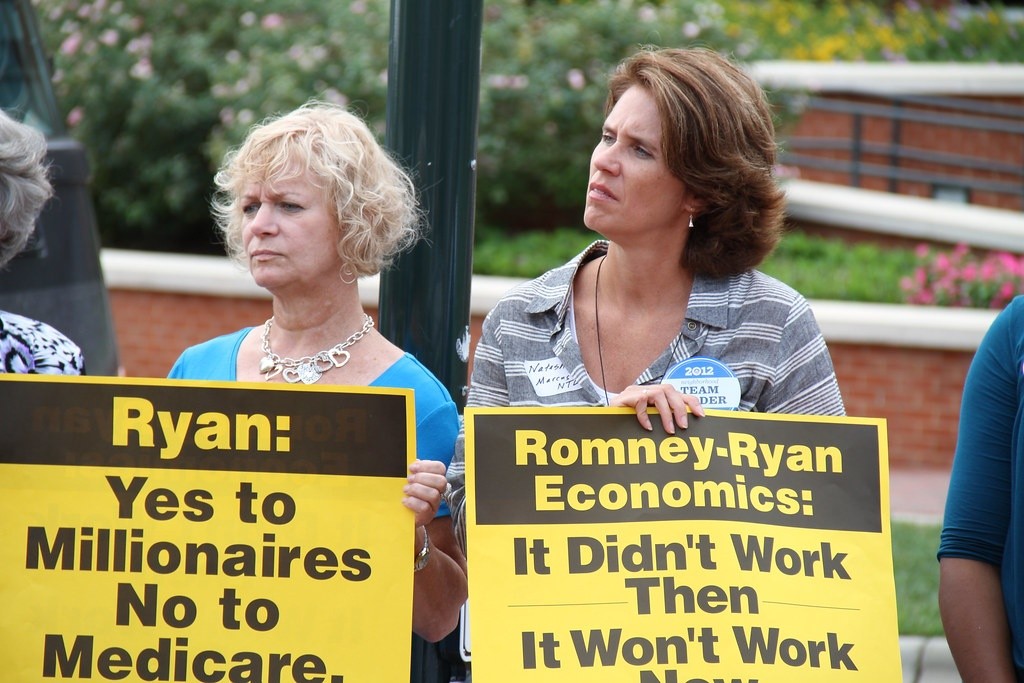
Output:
[441, 483, 452, 498]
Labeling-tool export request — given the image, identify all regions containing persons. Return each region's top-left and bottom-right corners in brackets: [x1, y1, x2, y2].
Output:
[0, 112, 86, 377]
[169, 103, 469, 683]
[449, 46, 845, 554]
[938, 294, 1024, 683]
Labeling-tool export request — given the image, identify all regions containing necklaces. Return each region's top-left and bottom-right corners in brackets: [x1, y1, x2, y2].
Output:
[595, 255, 682, 406]
[259, 312, 374, 384]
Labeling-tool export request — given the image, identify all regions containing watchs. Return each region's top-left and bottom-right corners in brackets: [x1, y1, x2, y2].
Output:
[415, 530, 430, 571]
